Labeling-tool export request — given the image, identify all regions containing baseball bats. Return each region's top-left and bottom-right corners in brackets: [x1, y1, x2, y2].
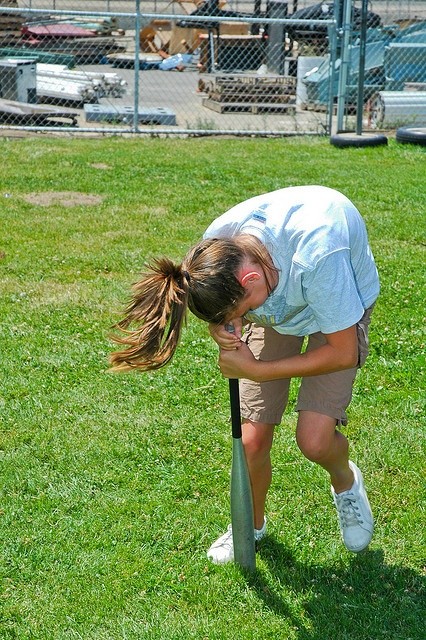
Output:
[225, 323, 256, 575]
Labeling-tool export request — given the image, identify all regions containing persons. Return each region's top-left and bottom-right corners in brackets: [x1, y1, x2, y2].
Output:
[104, 185, 380, 566]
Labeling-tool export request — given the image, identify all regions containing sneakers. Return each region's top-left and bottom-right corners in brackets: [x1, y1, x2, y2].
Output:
[205, 514, 268, 566]
[329, 460, 374, 553]
